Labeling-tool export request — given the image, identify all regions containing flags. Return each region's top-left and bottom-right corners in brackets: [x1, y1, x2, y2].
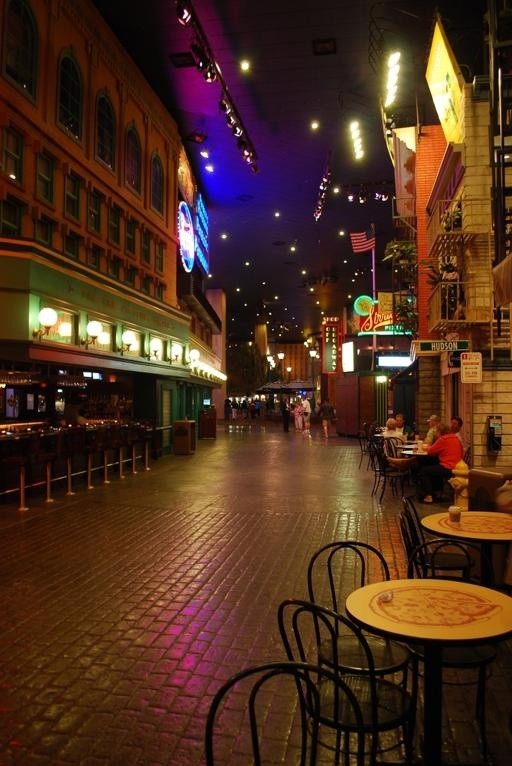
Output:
[349, 226, 376, 253]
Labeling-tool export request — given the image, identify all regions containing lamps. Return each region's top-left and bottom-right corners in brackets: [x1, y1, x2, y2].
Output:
[33, 307, 136, 352]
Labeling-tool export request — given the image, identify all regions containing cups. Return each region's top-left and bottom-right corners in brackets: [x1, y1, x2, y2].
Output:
[413, 441, 423, 453]
[449, 506, 461, 526]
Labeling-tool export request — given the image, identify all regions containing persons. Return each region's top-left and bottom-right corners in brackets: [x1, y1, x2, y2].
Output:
[417, 423, 463, 504]
[224, 396, 274, 422]
[301, 395, 311, 434]
[395, 414, 413, 433]
[425, 414, 441, 444]
[291, 402, 303, 433]
[450, 417, 466, 451]
[282, 398, 291, 433]
[383, 419, 404, 458]
[318, 397, 336, 438]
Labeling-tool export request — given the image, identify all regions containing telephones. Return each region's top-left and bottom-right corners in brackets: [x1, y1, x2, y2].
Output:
[487, 415, 503, 453]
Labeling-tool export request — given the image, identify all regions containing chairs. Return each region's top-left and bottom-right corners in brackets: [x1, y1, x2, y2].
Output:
[357, 421, 471, 505]
[205, 662, 365, 765]
